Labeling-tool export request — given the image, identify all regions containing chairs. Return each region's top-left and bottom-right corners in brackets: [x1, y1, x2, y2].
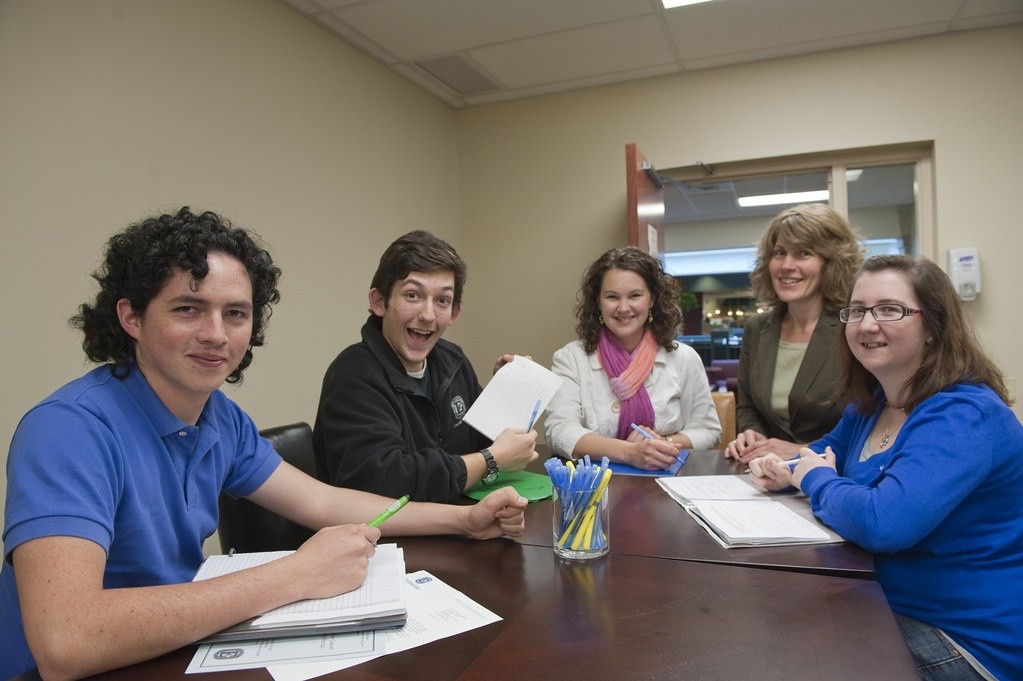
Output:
[711, 392, 740, 449]
[726, 378, 740, 404]
[213, 422, 323, 557]
[704, 367, 720, 391]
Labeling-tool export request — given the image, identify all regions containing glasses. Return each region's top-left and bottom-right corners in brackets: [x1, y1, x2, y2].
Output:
[837, 303, 923, 322]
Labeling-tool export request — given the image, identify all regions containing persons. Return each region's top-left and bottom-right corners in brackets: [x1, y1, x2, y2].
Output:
[752, 253, 1022, 680]
[2, 207, 529, 681]
[311, 229, 539, 503]
[724, 202, 868, 466]
[548, 245, 723, 467]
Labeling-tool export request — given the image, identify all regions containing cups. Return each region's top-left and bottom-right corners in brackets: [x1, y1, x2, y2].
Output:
[550, 482, 611, 560]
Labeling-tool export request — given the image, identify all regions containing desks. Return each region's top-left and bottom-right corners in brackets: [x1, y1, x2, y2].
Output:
[120, 533, 922, 680]
[447, 449, 877, 574]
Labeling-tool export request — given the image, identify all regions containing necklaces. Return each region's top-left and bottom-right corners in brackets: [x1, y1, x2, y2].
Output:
[878, 401, 907, 447]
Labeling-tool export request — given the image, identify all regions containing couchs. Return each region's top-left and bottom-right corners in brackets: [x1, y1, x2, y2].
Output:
[711, 360, 740, 386]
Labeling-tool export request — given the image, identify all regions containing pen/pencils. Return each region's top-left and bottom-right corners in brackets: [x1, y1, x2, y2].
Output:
[544, 455, 613, 552]
[631, 422, 688, 464]
[368, 494, 411, 530]
[528, 399, 540, 436]
